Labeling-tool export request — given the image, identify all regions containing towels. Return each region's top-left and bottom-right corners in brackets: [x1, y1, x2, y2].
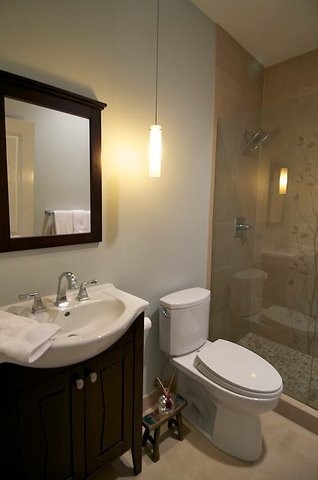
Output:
[0, 310, 62, 365]
[49, 209, 74, 236]
[73, 210, 91, 234]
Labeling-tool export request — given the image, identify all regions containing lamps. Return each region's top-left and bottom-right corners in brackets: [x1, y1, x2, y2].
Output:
[147, 124, 164, 180]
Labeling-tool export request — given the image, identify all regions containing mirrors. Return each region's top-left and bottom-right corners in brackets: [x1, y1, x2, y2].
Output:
[0, 69, 108, 254]
[266, 162, 288, 226]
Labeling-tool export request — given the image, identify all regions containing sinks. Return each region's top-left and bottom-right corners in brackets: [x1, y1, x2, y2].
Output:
[0, 283, 150, 368]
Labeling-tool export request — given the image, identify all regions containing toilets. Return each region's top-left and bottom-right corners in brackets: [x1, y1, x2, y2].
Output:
[158, 286, 284, 462]
[222, 269, 318, 334]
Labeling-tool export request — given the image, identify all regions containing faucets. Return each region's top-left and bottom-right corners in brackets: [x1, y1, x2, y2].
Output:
[53, 272, 78, 307]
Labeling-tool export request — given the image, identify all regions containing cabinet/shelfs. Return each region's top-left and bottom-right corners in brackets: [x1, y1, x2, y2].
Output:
[3, 314, 144, 476]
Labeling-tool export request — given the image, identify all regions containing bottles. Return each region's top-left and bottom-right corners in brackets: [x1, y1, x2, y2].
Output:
[158, 388, 176, 415]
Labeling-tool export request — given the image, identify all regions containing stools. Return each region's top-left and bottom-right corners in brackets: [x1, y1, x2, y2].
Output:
[144, 396, 188, 463]
